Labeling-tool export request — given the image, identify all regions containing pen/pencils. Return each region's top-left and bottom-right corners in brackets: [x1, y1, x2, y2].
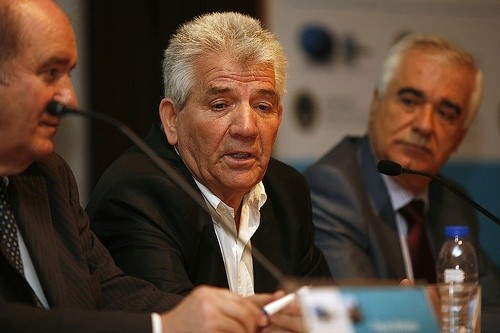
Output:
[261, 283, 312, 317]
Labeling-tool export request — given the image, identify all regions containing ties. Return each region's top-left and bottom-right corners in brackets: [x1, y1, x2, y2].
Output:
[397, 199, 437, 284]
[0, 176, 24, 278]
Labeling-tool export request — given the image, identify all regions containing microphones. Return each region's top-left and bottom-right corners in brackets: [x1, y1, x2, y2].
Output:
[377, 160, 500, 226]
[46, 101, 297, 295]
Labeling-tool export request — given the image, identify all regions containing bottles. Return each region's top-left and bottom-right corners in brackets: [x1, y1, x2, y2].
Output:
[435, 224, 482, 333]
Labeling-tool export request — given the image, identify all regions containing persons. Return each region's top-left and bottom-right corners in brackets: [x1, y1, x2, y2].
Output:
[86, 10, 314, 300]
[0, 0, 307, 333]
[303, 32, 500, 333]
